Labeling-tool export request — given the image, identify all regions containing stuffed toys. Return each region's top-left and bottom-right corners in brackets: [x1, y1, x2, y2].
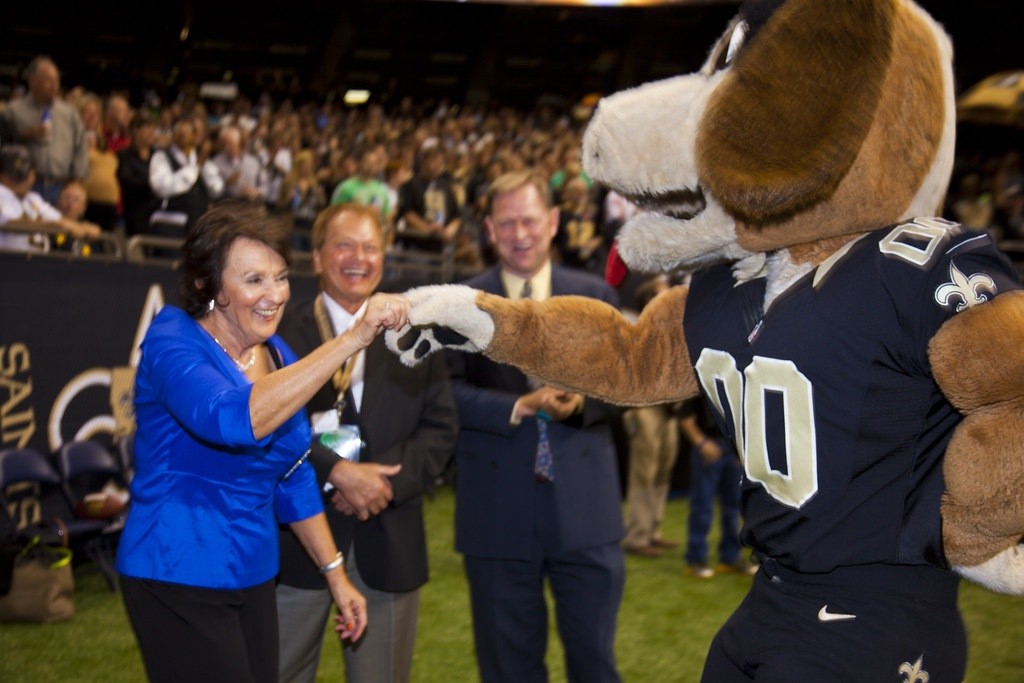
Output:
[383, 0, 1022, 683]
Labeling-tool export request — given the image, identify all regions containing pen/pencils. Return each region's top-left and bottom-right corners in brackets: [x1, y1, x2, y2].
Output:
[284, 448, 312, 482]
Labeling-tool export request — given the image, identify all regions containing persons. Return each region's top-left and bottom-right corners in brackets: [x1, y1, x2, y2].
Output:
[0, 54, 1024, 683]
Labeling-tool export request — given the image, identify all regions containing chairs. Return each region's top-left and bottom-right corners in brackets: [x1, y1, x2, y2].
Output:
[1, 440, 125, 595]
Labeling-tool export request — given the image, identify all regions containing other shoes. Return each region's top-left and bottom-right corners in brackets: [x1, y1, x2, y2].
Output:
[716, 560, 740, 573]
[684, 563, 715, 582]
[652, 536, 680, 549]
[627, 545, 661, 558]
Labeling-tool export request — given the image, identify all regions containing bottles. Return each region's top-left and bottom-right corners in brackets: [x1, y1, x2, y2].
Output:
[39, 109, 54, 146]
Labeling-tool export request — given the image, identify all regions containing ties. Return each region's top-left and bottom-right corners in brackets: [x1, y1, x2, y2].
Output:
[519, 282, 555, 482]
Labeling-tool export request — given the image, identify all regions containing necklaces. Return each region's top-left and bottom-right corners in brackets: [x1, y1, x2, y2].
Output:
[210, 331, 258, 371]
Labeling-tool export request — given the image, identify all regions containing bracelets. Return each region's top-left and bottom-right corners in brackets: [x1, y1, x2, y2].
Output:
[320, 552, 343, 572]
